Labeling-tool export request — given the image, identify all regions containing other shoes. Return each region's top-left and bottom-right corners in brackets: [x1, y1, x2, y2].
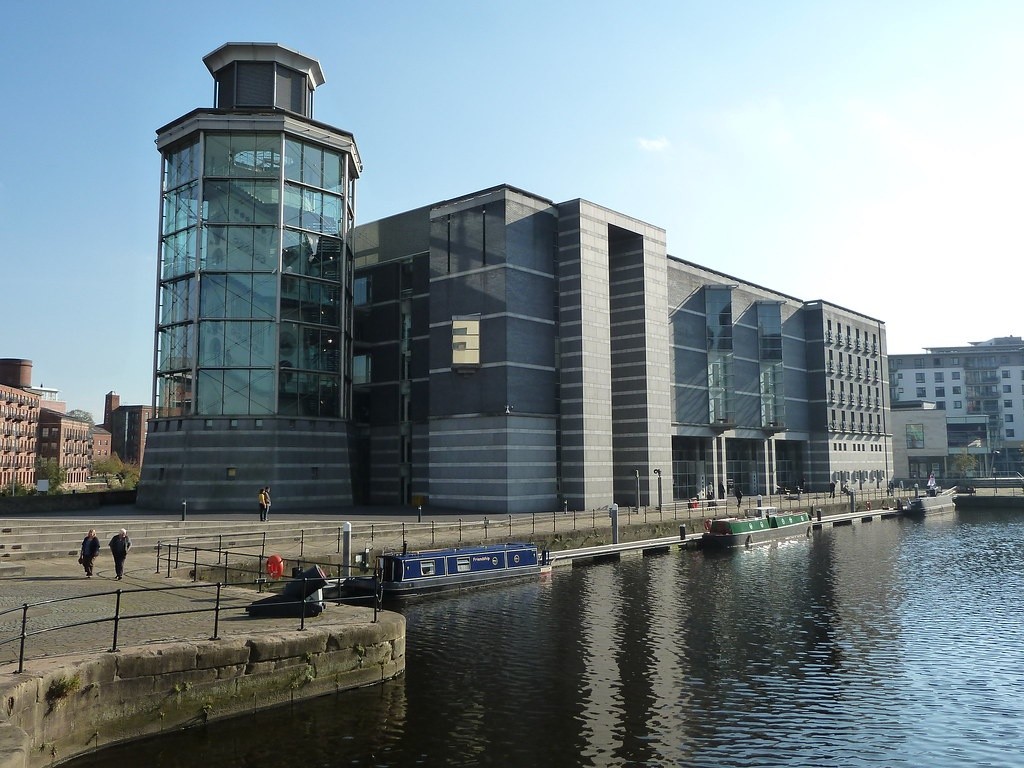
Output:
[265, 519, 269, 521]
[86, 574, 90, 578]
[260, 518, 264, 522]
[115, 576, 122, 580]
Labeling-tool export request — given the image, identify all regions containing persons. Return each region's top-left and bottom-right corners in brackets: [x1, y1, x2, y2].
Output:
[828, 480, 836, 498]
[888, 481, 894, 496]
[735, 487, 743, 507]
[264, 486, 272, 521]
[108, 528, 132, 580]
[708, 482, 713, 499]
[80, 529, 100, 576]
[259, 488, 266, 522]
[993, 466, 996, 477]
[718, 481, 725, 499]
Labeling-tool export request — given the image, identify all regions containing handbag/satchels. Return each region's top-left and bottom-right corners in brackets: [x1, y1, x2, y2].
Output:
[78, 551, 83, 564]
